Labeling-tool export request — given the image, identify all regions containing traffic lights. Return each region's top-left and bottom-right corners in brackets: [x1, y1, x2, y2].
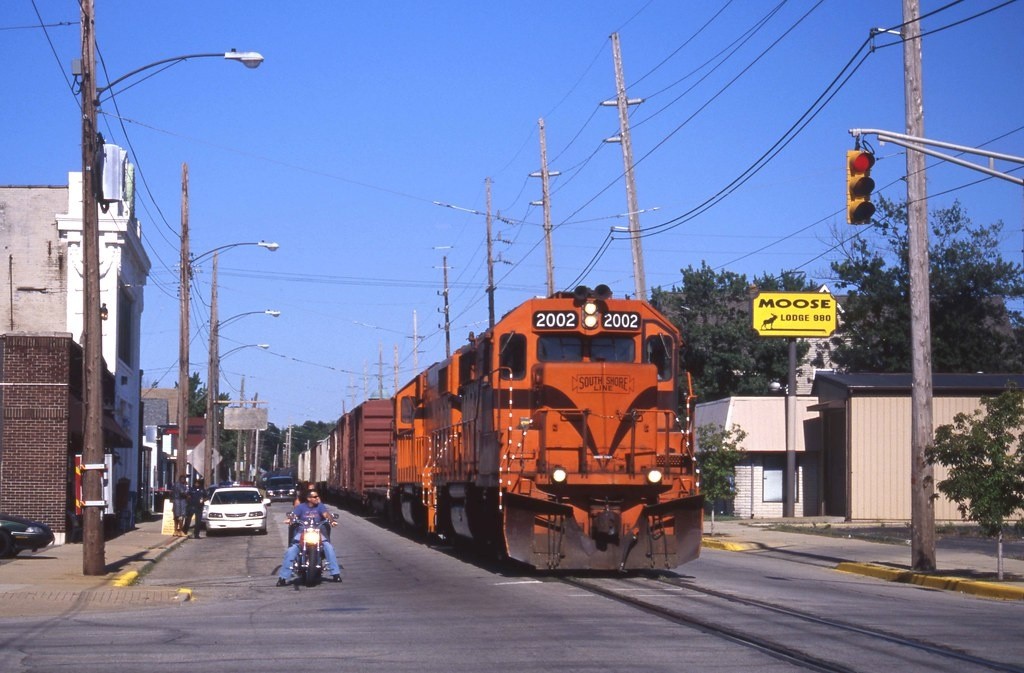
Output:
[845, 149, 876, 225]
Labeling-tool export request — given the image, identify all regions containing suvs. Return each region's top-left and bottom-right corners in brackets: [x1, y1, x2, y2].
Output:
[263, 475, 298, 502]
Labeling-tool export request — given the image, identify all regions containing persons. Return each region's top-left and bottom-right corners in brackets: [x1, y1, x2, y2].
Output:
[173, 476, 189, 536]
[288, 483, 322, 547]
[276, 489, 343, 587]
[183, 480, 210, 539]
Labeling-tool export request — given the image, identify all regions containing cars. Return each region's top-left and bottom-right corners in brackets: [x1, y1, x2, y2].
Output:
[204, 481, 271, 536]
[199, 480, 254, 523]
[0, 511, 54, 560]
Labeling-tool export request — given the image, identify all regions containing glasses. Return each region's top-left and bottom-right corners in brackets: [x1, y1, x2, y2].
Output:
[310, 495, 317, 499]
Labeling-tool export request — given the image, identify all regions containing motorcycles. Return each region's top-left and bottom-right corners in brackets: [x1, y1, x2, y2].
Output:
[278, 511, 340, 586]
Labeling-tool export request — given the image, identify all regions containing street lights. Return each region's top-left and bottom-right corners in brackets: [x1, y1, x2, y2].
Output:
[212, 343, 273, 486]
[80, 51, 264, 577]
[175, 241, 282, 485]
[202, 309, 284, 496]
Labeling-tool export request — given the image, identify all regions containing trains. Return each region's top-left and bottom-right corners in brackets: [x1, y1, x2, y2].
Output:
[292, 283, 707, 575]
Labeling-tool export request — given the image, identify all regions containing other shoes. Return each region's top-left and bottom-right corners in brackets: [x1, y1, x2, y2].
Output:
[174, 531, 178, 536]
[276, 577, 286, 587]
[177, 531, 187, 536]
[195, 535, 200, 538]
[332, 574, 342, 582]
